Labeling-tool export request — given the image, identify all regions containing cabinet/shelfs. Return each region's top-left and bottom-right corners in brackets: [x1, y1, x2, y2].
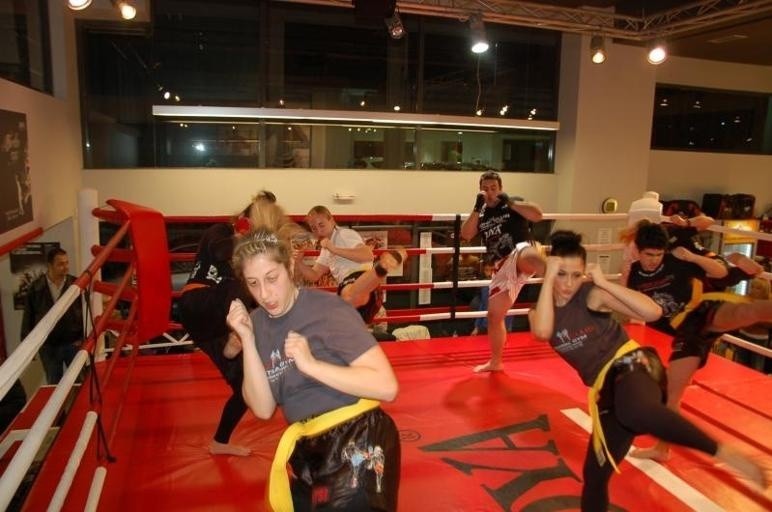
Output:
[704, 217, 760, 297]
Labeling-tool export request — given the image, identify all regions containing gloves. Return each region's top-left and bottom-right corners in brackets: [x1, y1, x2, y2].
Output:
[496, 193, 508, 203]
[474, 193, 485, 214]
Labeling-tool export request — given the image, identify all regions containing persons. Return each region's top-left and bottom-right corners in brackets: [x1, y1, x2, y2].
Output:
[294, 204, 407, 329]
[526, 229, 769, 511]
[460, 171, 549, 374]
[625, 223, 771, 463]
[662, 200, 705, 220]
[20, 249, 86, 385]
[448, 144, 463, 170]
[174, 190, 301, 457]
[265, 125, 309, 169]
[618, 216, 764, 293]
[223, 226, 402, 512]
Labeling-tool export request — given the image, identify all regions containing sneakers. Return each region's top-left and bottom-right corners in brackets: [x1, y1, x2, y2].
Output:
[377, 248, 408, 275]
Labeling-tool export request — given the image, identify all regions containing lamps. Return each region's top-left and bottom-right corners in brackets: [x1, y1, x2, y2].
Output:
[385, 5, 406, 39]
[466, 9, 490, 54]
[647, 38, 669, 65]
[116, 0, 137, 19]
[590, 25, 608, 64]
